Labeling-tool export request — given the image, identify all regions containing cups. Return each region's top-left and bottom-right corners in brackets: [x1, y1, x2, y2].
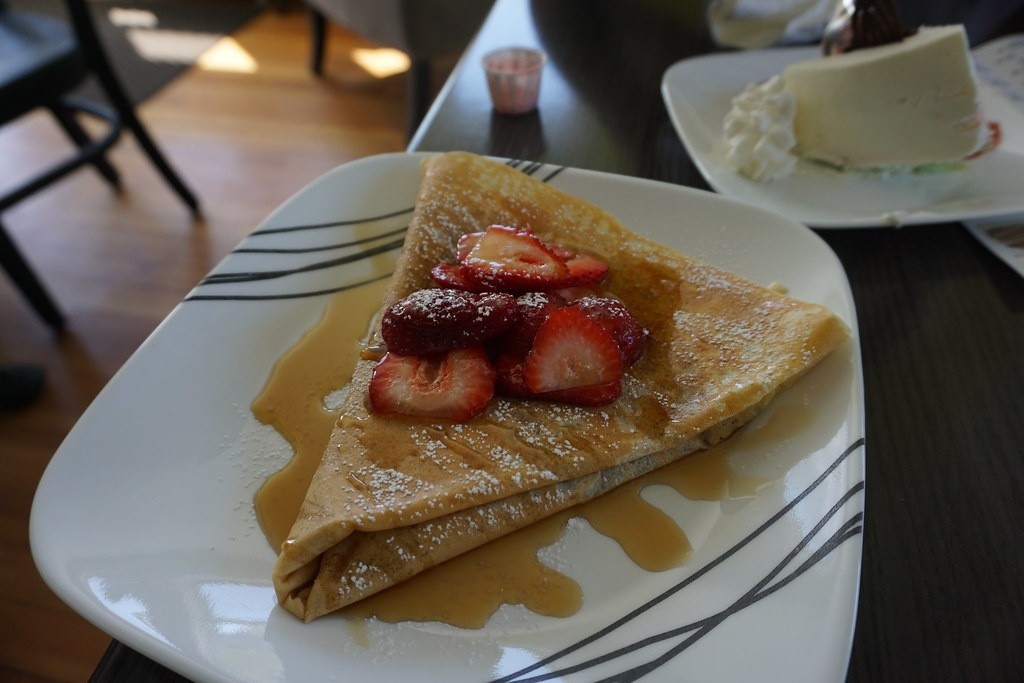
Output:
[480, 46, 547, 114]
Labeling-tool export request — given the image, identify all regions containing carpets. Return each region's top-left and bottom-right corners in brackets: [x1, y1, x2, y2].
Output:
[0, 0, 269, 115]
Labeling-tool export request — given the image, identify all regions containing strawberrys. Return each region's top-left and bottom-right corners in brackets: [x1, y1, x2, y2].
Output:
[368, 221, 645, 420]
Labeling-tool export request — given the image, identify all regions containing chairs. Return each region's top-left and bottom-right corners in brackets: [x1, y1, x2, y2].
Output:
[302, 0, 496, 143]
[0, 0, 199, 331]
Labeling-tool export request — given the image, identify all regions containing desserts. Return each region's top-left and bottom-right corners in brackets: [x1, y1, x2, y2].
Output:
[724, 22, 987, 179]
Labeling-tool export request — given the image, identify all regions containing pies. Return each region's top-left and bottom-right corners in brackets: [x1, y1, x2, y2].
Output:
[275, 147, 842, 617]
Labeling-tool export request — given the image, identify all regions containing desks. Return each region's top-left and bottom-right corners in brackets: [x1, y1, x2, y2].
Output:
[91, 0, 1024, 683]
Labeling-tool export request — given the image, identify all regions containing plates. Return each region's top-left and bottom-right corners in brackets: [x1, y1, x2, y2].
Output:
[28, 148, 867, 683]
[661, 45, 1024, 230]
